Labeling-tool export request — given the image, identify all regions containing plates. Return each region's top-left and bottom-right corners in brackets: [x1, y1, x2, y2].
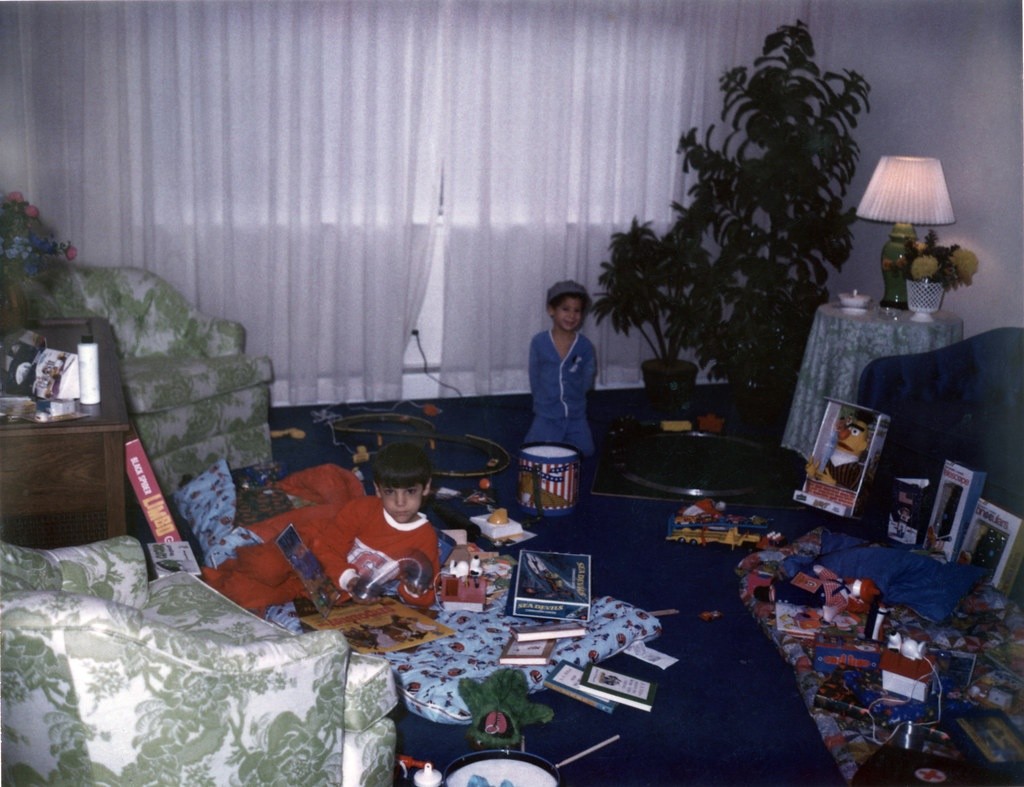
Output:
[842, 308, 867, 317]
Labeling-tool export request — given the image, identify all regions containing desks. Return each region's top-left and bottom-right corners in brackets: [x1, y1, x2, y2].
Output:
[780, 304, 964, 460]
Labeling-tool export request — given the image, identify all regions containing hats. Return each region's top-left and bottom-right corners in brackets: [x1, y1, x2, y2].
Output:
[547, 280, 588, 302]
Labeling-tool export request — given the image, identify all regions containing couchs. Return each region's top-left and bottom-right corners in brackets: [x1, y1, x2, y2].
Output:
[67, 266, 273, 507]
[0, 534, 400, 787]
[858, 327, 1024, 605]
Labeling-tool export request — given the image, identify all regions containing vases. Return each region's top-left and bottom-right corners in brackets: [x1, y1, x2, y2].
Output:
[907, 276, 944, 312]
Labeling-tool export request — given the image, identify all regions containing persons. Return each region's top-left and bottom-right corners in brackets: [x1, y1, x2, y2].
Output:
[805, 409, 875, 494]
[200, 441, 441, 612]
[524, 281, 596, 459]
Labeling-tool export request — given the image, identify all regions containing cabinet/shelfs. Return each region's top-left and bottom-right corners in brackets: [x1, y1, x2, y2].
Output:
[0, 317, 129, 551]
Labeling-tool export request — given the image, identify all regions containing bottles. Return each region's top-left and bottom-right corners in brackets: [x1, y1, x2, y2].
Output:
[871, 608, 888, 641]
[413, 762, 442, 787]
[76, 334, 101, 405]
[863, 594, 881, 641]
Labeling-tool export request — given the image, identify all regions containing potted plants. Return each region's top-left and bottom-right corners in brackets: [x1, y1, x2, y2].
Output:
[590, 16, 871, 407]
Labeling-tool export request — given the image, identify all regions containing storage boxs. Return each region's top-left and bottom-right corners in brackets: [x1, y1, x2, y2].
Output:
[923, 460, 1024, 596]
[790, 397, 891, 520]
[124, 419, 202, 580]
[512, 550, 591, 623]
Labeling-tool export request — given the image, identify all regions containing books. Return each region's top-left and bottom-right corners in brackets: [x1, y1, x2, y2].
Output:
[499, 621, 587, 666]
[442, 575, 487, 612]
[814, 664, 954, 746]
[543, 658, 656, 712]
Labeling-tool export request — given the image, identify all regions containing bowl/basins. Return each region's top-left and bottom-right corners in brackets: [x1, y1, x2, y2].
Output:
[442, 750, 561, 787]
[839, 292, 871, 307]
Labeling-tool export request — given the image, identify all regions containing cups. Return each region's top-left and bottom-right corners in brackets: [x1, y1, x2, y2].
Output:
[894, 723, 925, 751]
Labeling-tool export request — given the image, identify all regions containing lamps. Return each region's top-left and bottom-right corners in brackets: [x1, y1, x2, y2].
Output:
[856, 156, 955, 310]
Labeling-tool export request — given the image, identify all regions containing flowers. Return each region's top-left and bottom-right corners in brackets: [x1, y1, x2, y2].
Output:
[0, 191, 78, 286]
[903, 230, 979, 291]
